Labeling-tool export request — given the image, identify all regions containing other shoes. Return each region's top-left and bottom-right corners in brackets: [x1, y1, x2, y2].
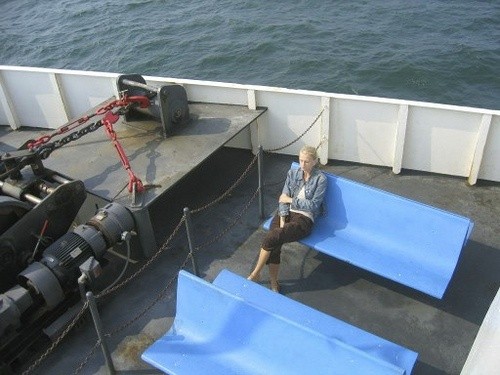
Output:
[272, 283, 281, 293]
[247, 273, 262, 283]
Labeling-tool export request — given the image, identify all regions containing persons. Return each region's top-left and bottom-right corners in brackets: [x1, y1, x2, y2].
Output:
[245, 145, 329, 295]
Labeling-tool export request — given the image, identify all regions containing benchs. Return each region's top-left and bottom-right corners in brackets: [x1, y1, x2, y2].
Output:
[140, 265, 420, 374]
[260, 162, 474, 300]
